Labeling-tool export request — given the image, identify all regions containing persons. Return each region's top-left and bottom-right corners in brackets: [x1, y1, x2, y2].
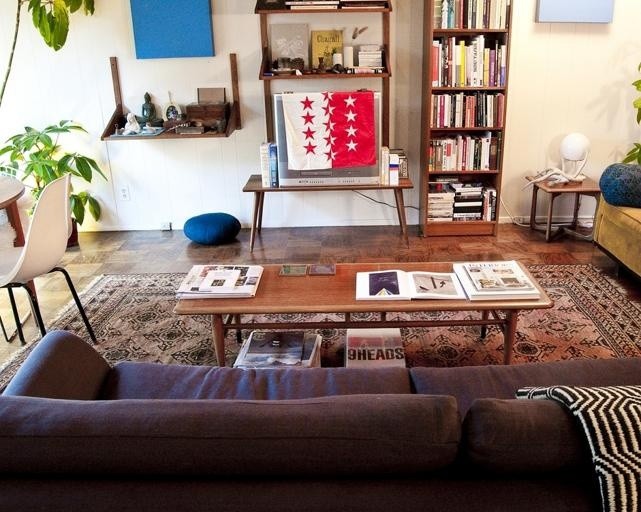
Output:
[125, 114, 140, 133]
[140, 94, 160, 123]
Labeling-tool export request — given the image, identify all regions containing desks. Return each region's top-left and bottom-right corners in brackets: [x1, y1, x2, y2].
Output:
[0, 173, 45, 326]
[0, 173, 38, 300]
[524, 173, 601, 244]
[242, 172, 416, 254]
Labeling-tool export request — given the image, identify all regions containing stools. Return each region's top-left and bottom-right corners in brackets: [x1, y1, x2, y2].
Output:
[184, 212, 241, 245]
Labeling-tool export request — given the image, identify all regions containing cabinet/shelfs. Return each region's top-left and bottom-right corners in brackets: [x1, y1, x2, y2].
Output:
[417, 0, 514, 238]
[254, 0, 395, 81]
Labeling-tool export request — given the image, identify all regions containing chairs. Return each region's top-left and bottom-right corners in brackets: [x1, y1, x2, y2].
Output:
[0, 172, 100, 348]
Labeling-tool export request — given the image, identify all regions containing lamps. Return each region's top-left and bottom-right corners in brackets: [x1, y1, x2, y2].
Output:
[559, 132, 591, 184]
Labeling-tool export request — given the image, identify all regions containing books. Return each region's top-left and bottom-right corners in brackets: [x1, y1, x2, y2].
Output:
[233, 330, 323, 368]
[381, 145, 408, 186]
[311, 30, 345, 71]
[271, 24, 310, 71]
[345, 329, 407, 369]
[354, 43, 383, 68]
[179, 264, 265, 298]
[357, 270, 467, 300]
[428, 0, 513, 220]
[283, 0, 387, 10]
[261, 142, 279, 189]
[454, 259, 541, 302]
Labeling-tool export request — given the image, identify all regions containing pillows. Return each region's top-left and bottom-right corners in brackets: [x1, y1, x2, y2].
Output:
[599, 164, 641, 208]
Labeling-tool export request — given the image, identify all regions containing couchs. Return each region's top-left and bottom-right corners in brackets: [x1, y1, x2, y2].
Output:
[593, 163, 641, 280]
[0, 331, 641, 511]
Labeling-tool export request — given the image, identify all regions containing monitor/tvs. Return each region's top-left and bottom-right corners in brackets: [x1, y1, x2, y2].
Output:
[273, 92, 382, 188]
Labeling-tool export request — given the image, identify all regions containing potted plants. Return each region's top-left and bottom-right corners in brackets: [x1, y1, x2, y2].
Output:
[0, 119, 109, 247]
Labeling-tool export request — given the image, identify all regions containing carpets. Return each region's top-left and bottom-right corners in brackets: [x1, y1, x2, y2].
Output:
[0, 260, 641, 393]
